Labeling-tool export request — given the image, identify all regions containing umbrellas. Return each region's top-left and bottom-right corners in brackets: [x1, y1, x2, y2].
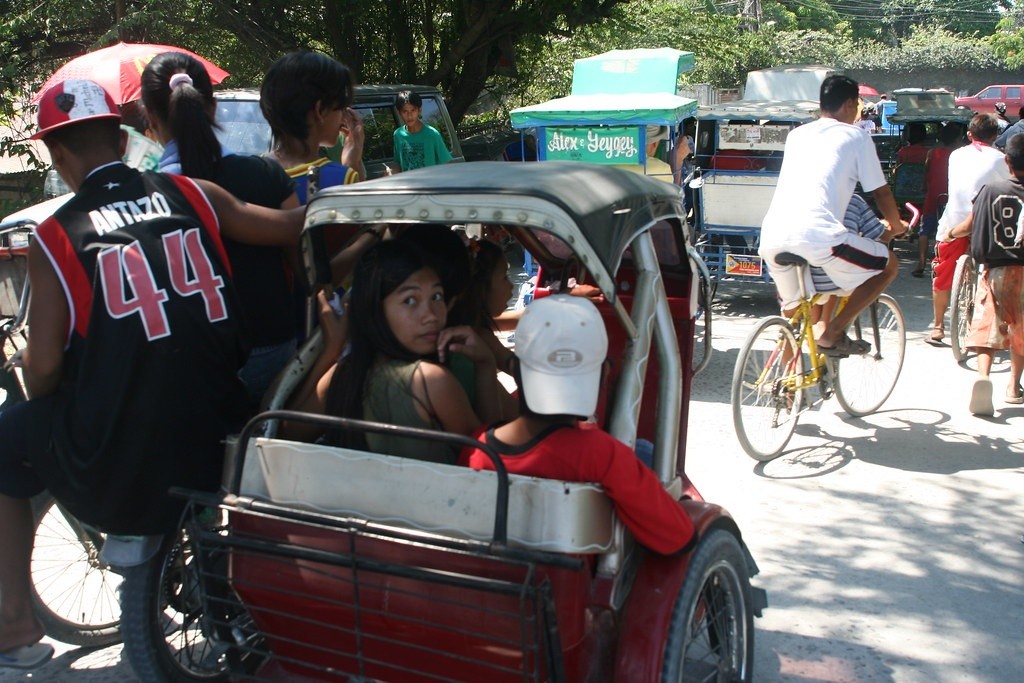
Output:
[859, 86, 878, 96]
[29, 42, 231, 106]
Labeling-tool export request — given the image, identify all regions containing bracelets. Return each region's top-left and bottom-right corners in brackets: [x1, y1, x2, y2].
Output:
[948, 228, 956, 239]
[368, 227, 383, 239]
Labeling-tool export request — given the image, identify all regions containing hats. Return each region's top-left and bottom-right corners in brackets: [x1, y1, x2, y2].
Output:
[29, 79, 124, 140]
[515, 293, 608, 417]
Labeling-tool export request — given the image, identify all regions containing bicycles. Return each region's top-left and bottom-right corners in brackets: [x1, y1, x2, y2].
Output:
[731, 202, 922, 464]
[948, 230, 983, 362]
[0, 256, 130, 648]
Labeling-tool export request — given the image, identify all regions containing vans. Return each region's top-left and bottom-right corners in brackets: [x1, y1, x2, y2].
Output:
[213, 84, 466, 187]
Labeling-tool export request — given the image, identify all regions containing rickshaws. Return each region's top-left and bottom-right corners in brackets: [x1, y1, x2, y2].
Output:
[117, 150, 773, 683]
[513, 47, 857, 317]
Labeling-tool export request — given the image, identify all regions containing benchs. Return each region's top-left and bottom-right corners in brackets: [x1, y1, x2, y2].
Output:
[219, 434, 614, 683]
[699, 171, 780, 233]
[894, 161, 928, 203]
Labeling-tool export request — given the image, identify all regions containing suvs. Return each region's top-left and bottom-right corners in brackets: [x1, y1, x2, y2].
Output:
[954, 84, 1024, 117]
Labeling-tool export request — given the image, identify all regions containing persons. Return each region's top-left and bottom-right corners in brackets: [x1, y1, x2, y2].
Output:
[895, 122, 968, 276]
[992, 102, 1024, 152]
[615, 116, 783, 269]
[385, 90, 453, 176]
[853, 94, 888, 134]
[396, 223, 520, 424]
[923, 113, 1014, 345]
[259, 54, 367, 260]
[276, 240, 502, 463]
[758, 75, 908, 415]
[120, 104, 162, 170]
[325, 130, 366, 181]
[141, 51, 387, 416]
[0, 78, 307, 669]
[458, 294, 698, 560]
[945, 132, 1024, 417]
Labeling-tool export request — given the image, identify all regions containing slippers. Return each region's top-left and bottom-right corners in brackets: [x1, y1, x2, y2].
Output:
[817, 331, 872, 354]
[925, 334, 943, 345]
[911, 269, 922, 276]
[1005, 385, 1024, 404]
[970, 379, 994, 416]
[0, 643, 54, 668]
